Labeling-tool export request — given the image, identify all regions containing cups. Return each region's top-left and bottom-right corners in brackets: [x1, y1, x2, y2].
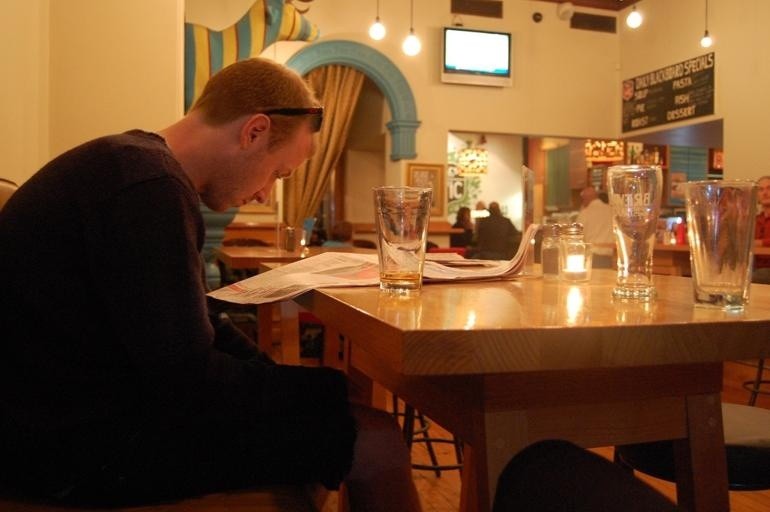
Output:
[271, 212, 319, 260]
[558, 235, 599, 286]
[682, 176, 759, 319]
[605, 163, 669, 305]
[370, 184, 436, 293]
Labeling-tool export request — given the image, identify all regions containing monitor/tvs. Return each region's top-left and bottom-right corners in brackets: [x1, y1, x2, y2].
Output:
[441, 25, 516, 88]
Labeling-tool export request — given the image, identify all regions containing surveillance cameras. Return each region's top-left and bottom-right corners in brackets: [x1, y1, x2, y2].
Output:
[533, 12, 543, 22]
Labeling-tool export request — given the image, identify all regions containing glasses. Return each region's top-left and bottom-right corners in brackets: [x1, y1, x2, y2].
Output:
[263, 103, 325, 133]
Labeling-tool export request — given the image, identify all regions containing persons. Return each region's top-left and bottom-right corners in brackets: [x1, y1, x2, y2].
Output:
[0, 56, 424, 512]
[448, 205, 473, 248]
[575, 186, 618, 270]
[492, 439, 681, 511]
[317, 221, 355, 247]
[753, 176, 770, 245]
[473, 202, 519, 256]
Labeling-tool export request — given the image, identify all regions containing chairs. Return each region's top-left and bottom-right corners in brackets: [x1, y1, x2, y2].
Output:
[615, 359, 770, 492]
[391, 392, 464, 483]
[0, 178, 320, 512]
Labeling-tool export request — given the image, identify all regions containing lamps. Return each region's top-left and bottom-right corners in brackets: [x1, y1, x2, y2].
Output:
[624, 0, 643, 28]
[701, 1, 711, 47]
[400, 0, 422, 56]
[369, 0, 386, 41]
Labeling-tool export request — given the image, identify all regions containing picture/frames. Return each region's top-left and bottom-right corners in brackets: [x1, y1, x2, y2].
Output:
[406, 161, 446, 219]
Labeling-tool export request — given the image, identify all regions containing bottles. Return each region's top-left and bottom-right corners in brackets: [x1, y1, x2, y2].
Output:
[540, 222, 586, 281]
